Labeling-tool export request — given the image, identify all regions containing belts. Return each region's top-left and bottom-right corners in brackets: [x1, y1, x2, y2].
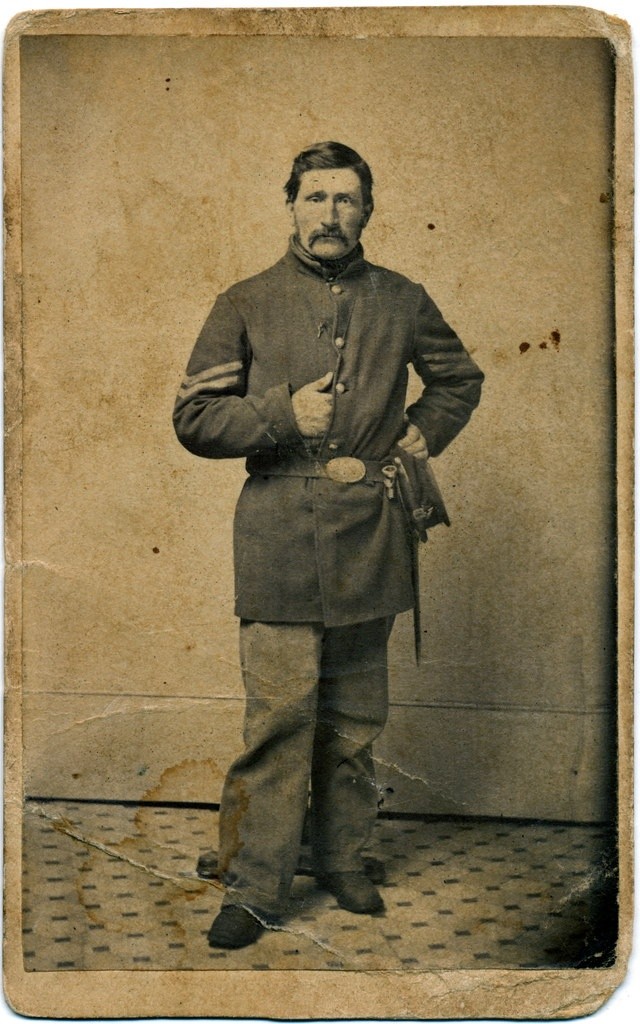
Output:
[244, 457, 398, 484]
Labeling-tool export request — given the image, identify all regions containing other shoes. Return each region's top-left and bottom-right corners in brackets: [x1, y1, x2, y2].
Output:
[207, 903, 262, 948]
[315, 869, 385, 914]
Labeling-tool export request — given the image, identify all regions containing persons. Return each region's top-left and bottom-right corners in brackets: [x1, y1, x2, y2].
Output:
[173, 142, 484, 951]
